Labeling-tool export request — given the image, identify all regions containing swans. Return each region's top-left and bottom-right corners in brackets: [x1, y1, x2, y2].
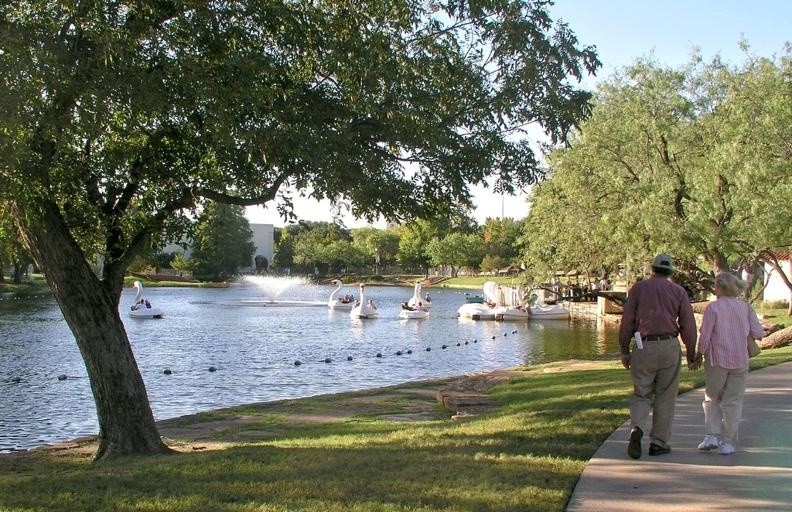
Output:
[327, 279, 379, 317]
[399, 280, 432, 319]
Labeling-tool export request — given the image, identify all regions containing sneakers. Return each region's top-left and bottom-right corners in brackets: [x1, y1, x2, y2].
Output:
[718, 441, 736, 455]
[697, 435, 718, 450]
[649, 442, 670, 456]
[628, 426, 642, 459]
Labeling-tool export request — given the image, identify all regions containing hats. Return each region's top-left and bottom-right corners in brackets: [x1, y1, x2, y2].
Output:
[651, 254, 673, 269]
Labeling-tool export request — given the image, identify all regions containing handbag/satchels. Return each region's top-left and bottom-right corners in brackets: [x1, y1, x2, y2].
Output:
[747, 334, 761, 358]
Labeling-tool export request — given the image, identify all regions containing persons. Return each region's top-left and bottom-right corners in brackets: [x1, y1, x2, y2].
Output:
[401, 292, 431, 312]
[618, 252, 697, 460]
[691, 272, 765, 455]
[130, 298, 151, 311]
[555, 274, 615, 303]
[339, 294, 355, 304]
[354, 297, 379, 311]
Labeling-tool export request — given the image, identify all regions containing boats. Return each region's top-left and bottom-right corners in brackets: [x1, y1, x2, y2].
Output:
[328, 279, 569, 321]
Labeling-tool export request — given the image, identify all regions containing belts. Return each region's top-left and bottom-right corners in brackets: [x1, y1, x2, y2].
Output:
[642, 333, 676, 341]
